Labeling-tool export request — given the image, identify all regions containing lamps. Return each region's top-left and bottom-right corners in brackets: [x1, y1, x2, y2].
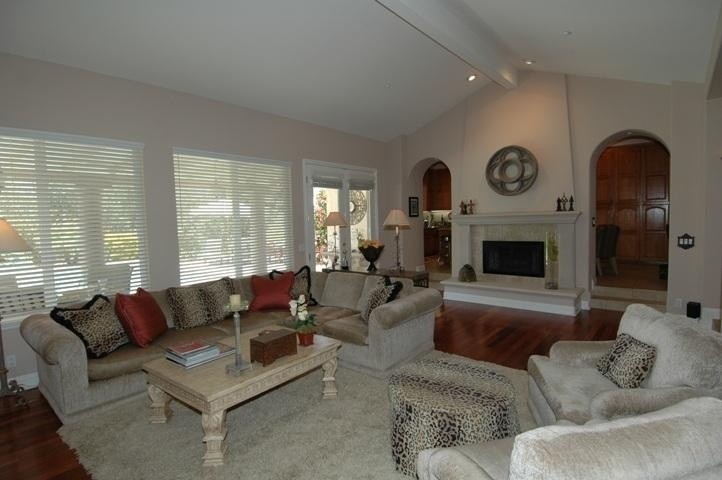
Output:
[323, 212, 348, 269]
[383, 209, 410, 271]
[0, 218, 34, 418]
[678, 233, 695, 250]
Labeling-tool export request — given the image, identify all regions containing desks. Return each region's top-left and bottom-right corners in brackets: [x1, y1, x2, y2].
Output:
[322, 269, 429, 288]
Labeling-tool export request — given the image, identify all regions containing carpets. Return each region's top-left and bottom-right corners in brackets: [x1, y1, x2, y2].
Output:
[659, 264, 668, 280]
[56, 364, 539, 480]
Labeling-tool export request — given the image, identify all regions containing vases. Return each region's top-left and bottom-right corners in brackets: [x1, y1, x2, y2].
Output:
[296, 331, 317, 346]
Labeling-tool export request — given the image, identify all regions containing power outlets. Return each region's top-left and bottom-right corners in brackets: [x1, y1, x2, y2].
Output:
[7, 355, 16, 369]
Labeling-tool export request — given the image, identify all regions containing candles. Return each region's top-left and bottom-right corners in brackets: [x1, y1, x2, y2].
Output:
[230, 294, 241, 309]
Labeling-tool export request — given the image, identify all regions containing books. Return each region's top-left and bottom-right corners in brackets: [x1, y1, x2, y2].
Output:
[165, 335, 236, 370]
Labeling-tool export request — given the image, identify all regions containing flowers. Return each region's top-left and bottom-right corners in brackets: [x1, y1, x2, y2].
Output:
[289, 294, 318, 331]
[356, 229, 385, 271]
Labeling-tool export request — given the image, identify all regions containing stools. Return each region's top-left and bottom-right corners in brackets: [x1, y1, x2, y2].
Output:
[387, 358, 521, 479]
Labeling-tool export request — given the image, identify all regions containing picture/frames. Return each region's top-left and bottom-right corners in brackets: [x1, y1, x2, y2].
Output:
[409, 197, 419, 217]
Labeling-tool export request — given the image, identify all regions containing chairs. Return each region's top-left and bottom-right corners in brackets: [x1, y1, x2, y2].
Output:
[595, 223, 621, 276]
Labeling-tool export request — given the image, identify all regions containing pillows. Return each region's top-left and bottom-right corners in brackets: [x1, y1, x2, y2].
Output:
[115, 286, 169, 348]
[166, 275, 235, 329]
[597, 333, 657, 388]
[359, 274, 403, 324]
[52, 294, 128, 359]
[271, 265, 318, 306]
[250, 271, 298, 311]
